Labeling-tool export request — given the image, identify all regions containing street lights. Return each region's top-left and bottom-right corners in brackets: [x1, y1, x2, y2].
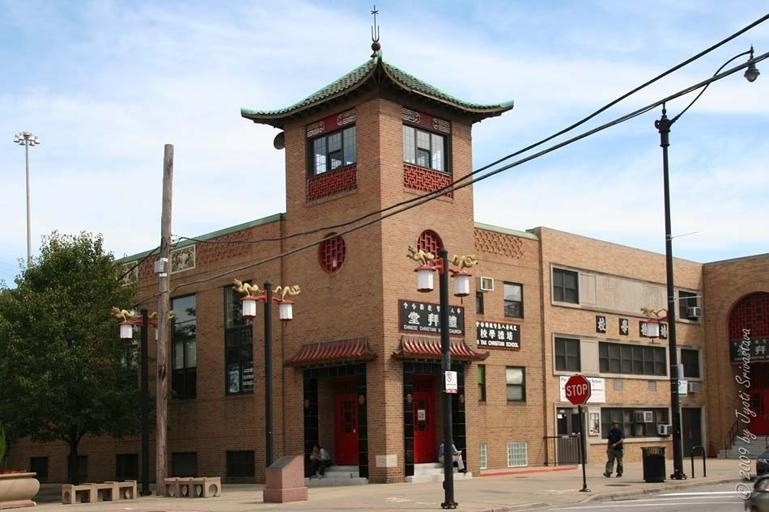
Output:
[654, 45, 761, 481]
[231, 278, 301, 468]
[111, 307, 176, 496]
[405, 245, 478, 509]
[13, 130, 40, 268]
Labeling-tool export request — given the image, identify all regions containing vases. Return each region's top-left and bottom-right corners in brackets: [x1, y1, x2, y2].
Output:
[0, 472, 40, 510]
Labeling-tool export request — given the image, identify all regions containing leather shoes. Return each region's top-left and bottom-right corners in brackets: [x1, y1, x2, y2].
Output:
[458, 469, 467, 473]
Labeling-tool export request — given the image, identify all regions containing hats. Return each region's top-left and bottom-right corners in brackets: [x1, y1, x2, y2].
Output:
[611, 419, 619, 423]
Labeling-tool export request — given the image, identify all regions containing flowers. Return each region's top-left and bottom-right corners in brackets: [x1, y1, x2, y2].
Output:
[0, 466, 28, 475]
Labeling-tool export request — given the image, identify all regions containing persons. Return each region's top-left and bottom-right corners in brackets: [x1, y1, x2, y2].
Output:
[437, 440, 467, 474]
[308, 443, 334, 476]
[603, 421, 625, 478]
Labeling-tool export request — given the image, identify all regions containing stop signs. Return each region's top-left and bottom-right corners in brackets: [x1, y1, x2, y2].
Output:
[565, 374, 591, 405]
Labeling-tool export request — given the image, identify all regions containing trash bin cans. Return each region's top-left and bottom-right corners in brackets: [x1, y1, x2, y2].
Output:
[639, 446, 667, 483]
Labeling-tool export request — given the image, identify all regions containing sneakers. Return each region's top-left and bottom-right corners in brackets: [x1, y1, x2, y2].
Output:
[604, 473, 610, 477]
[616, 474, 622, 478]
